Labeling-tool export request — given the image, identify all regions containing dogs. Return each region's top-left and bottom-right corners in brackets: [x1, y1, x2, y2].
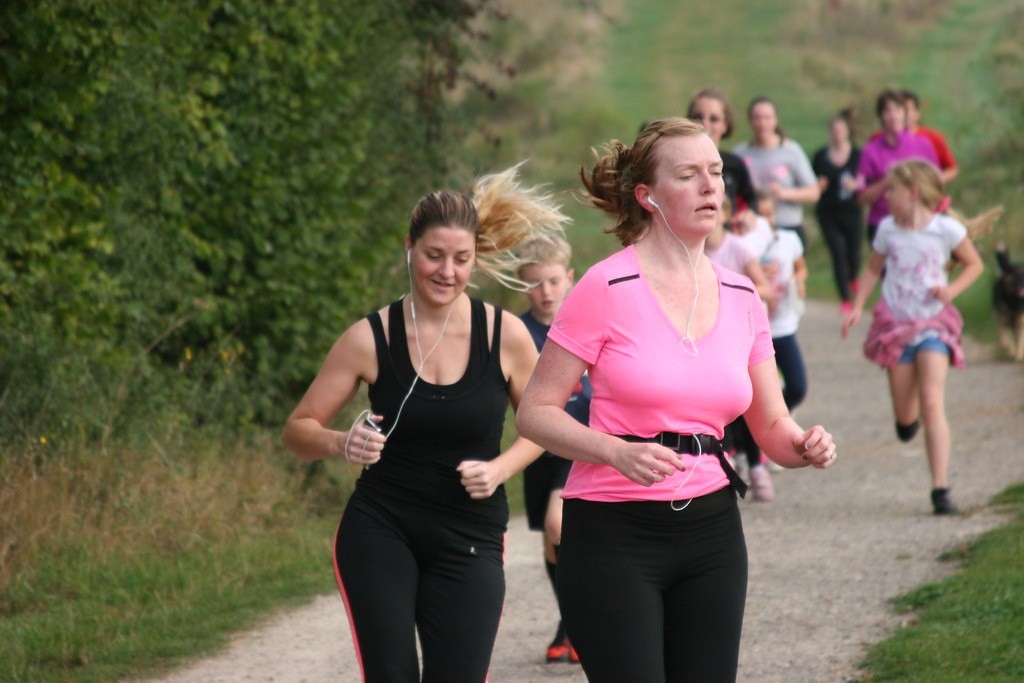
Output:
[992, 244, 1024, 361]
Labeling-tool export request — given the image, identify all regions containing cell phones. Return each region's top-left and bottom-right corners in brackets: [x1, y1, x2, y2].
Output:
[363, 419, 381, 469]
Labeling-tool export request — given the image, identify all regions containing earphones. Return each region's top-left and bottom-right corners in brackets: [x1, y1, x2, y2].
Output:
[648, 195, 660, 209]
[407, 250, 412, 264]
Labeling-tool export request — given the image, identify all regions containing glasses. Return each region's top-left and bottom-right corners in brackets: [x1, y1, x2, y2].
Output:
[694, 112, 720, 123]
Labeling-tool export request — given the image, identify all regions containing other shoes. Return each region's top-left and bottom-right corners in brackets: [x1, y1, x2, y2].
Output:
[750, 467, 772, 502]
[934, 494, 957, 514]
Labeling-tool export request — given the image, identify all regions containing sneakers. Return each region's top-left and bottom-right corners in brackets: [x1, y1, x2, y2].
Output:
[546, 615, 579, 669]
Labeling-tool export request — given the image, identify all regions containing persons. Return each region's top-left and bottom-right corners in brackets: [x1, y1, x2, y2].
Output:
[841, 157, 983, 518]
[513, 119, 839, 682]
[282, 160, 571, 683]
[688, 87, 958, 502]
[512, 232, 591, 662]
